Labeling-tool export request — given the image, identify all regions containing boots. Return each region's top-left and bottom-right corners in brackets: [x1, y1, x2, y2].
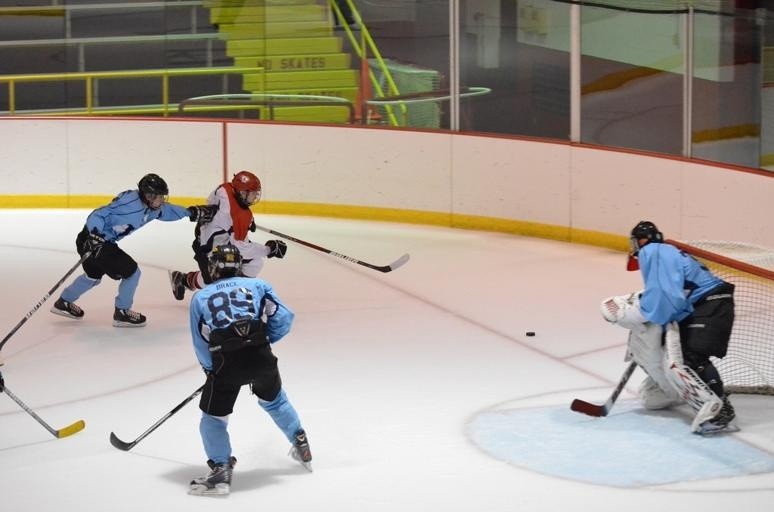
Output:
[702, 394, 736, 431]
[189, 457, 237, 485]
[290, 427, 312, 463]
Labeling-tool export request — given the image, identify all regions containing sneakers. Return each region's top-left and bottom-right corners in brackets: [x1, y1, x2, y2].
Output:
[54, 297, 84, 317]
[171, 271, 185, 301]
[113, 306, 146, 324]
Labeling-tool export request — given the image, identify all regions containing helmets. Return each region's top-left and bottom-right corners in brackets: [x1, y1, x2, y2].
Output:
[138, 174, 169, 211]
[207, 245, 244, 282]
[629, 220, 664, 258]
[233, 171, 262, 209]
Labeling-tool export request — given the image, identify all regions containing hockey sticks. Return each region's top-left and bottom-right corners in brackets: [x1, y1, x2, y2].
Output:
[571, 360, 637, 419]
[255, 224, 411, 273]
[110, 383, 206, 451]
[2, 385, 84, 438]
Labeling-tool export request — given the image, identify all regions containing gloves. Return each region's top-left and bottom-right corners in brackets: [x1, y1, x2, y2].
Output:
[189, 204, 217, 223]
[265, 239, 287, 258]
[84, 227, 107, 260]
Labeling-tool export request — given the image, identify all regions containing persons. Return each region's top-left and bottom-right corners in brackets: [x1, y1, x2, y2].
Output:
[172, 170, 287, 302]
[55, 174, 215, 324]
[190, 245, 312, 488]
[599, 221, 736, 430]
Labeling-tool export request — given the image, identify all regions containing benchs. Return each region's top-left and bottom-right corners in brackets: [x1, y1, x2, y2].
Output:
[0, 1, 410, 130]
